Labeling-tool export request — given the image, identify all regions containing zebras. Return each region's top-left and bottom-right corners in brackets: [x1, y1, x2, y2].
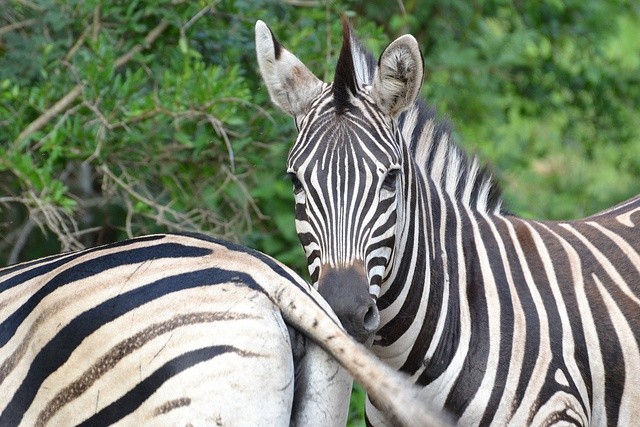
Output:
[0, 229, 463, 427]
[254, 11, 640, 427]
